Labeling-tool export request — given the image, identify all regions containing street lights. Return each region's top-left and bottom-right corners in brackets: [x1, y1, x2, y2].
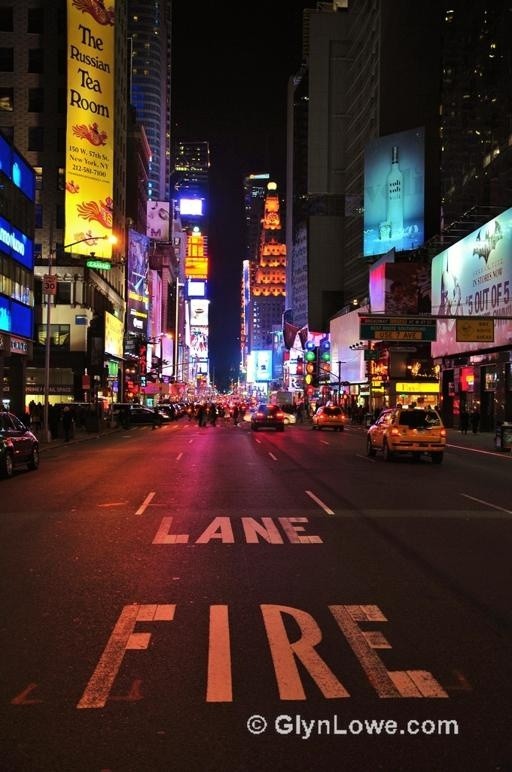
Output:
[41, 234, 118, 444]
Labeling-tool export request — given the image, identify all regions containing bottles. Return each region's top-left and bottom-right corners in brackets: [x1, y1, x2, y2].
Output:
[385, 144, 404, 238]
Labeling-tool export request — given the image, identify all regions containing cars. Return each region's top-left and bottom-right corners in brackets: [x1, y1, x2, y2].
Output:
[157, 402, 187, 420]
[243, 408, 297, 426]
[118, 407, 171, 425]
[0, 410, 40, 480]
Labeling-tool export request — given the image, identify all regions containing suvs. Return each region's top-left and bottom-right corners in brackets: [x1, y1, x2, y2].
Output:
[364, 406, 448, 465]
[311, 406, 347, 432]
[250, 403, 285, 432]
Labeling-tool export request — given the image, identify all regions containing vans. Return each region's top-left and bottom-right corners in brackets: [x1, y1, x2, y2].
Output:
[108, 402, 155, 417]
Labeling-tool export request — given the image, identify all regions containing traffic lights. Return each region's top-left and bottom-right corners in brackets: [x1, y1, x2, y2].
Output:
[304, 348, 320, 387]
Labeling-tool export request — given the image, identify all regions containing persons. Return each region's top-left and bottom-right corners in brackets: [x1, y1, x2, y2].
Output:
[460, 408, 469, 435]
[118, 407, 130, 426]
[279, 401, 315, 423]
[151, 406, 160, 430]
[470, 408, 480, 433]
[397, 401, 439, 413]
[0, 400, 89, 443]
[169, 402, 256, 427]
[344, 403, 369, 425]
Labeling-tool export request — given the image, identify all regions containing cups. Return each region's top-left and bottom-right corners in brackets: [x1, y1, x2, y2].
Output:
[378, 221, 392, 241]
